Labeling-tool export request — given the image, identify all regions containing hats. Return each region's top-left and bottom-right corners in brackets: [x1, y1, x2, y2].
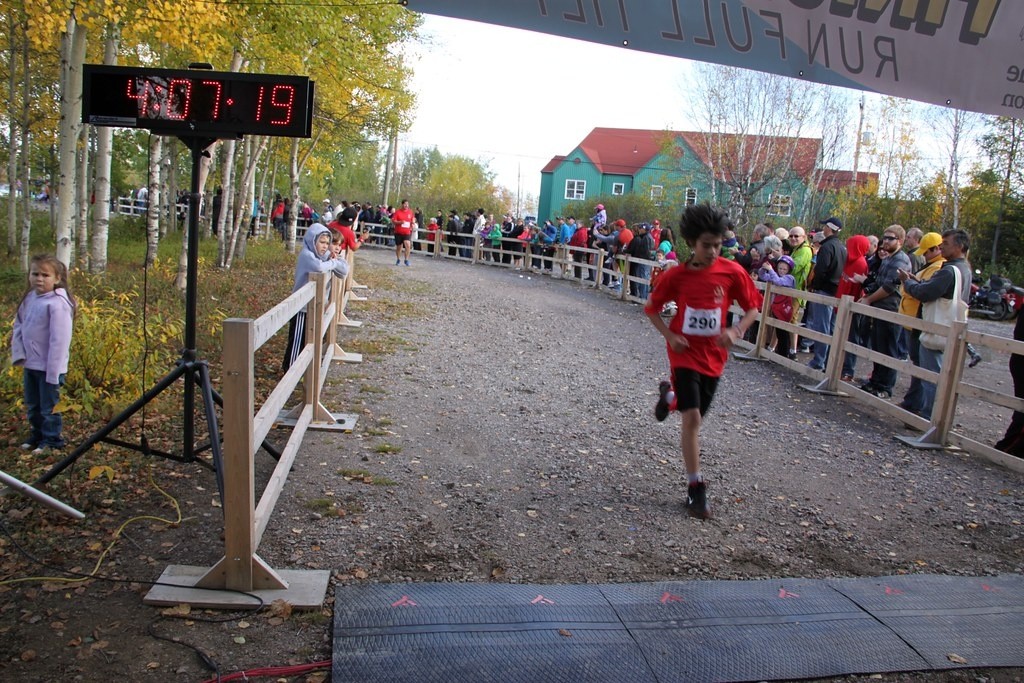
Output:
[665, 252, 676, 260]
[593, 204, 604, 210]
[820, 217, 842, 232]
[638, 220, 660, 230]
[556, 216, 566, 220]
[450, 210, 458, 214]
[567, 216, 575, 220]
[913, 233, 942, 256]
[323, 199, 330, 204]
[338, 208, 357, 226]
[614, 219, 626, 227]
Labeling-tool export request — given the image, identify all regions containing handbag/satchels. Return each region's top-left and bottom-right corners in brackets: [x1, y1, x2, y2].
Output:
[918, 265, 969, 351]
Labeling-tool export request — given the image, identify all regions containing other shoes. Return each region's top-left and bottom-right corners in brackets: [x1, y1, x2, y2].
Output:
[612, 281, 620, 286]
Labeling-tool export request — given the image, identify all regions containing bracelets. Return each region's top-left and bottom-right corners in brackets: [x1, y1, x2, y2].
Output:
[359, 237, 363, 242]
[735, 325, 742, 334]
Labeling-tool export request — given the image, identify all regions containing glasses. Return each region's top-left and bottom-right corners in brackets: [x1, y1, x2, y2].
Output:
[883, 235, 899, 241]
[789, 234, 803, 238]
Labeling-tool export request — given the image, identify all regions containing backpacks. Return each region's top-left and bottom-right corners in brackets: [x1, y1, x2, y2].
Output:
[771, 295, 794, 322]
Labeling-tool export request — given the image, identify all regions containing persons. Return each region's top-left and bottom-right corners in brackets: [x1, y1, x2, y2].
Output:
[898, 228, 972, 431]
[325, 225, 344, 254]
[993, 303, 1024, 458]
[122, 184, 222, 236]
[392, 199, 415, 266]
[359, 201, 424, 251]
[644, 201, 765, 517]
[327, 207, 368, 251]
[10, 254, 76, 455]
[247, 193, 360, 242]
[10, 174, 50, 202]
[282, 223, 348, 384]
[427, 208, 677, 305]
[719, 216, 983, 414]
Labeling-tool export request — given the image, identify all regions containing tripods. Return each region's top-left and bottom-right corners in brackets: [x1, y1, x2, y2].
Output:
[35, 139, 285, 516]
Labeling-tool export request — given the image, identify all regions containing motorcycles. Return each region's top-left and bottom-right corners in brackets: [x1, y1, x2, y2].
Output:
[968, 269, 1024, 321]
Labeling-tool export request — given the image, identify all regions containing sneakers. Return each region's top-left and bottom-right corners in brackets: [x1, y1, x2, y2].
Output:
[654, 381, 673, 422]
[868, 388, 889, 398]
[841, 373, 853, 381]
[404, 260, 411, 267]
[682, 476, 713, 520]
[788, 353, 799, 361]
[969, 355, 981, 367]
[861, 382, 874, 391]
[396, 259, 402, 266]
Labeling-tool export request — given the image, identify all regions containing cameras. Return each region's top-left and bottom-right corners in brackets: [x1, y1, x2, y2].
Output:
[862, 277, 880, 294]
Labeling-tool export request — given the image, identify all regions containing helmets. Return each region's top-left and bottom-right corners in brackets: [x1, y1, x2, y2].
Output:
[778, 255, 794, 274]
